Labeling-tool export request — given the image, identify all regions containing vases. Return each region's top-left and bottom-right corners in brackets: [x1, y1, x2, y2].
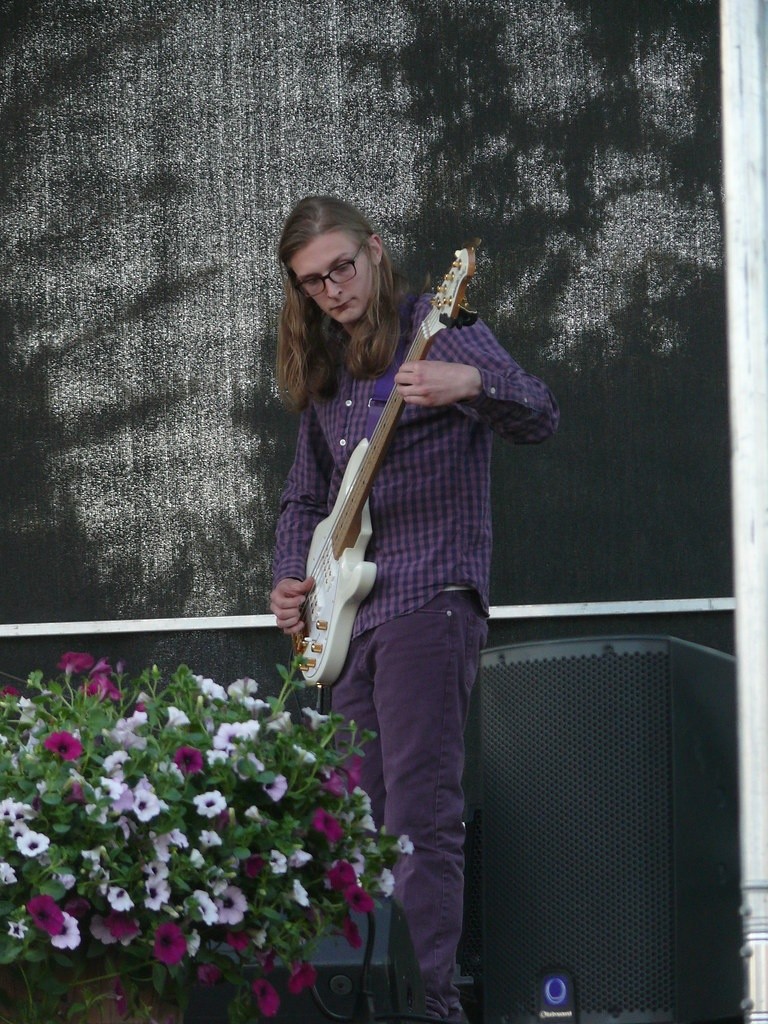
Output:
[0, 948, 192, 1024]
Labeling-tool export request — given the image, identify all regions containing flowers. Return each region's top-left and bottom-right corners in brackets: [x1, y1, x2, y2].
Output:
[0, 653, 415, 1024]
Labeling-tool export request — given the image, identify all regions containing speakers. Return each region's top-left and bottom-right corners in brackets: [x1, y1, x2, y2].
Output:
[457, 635, 750, 1024]
[181, 891, 427, 1024]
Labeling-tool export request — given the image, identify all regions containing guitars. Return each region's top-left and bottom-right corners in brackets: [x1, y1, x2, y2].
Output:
[291, 236, 482, 690]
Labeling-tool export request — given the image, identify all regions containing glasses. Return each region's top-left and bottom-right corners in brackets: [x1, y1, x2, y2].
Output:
[297, 241, 363, 298]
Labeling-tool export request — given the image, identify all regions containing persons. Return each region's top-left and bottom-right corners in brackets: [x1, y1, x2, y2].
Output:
[268, 197, 559, 1024]
